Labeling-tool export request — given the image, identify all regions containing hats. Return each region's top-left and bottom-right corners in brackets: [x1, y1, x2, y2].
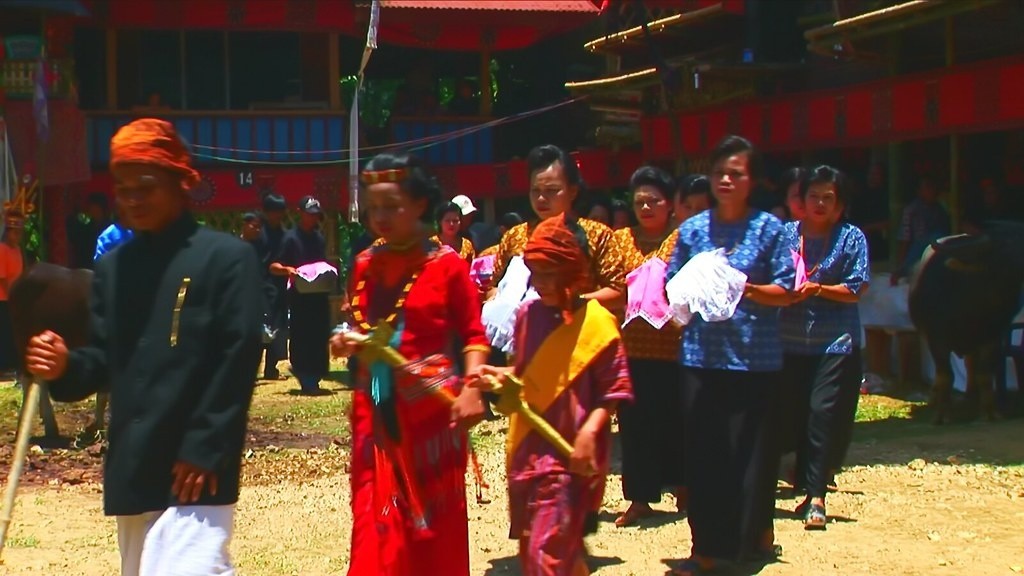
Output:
[451, 194, 477, 216]
[108, 117, 207, 193]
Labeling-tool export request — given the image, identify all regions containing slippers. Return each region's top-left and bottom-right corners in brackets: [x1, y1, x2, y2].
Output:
[827, 475, 838, 491]
[615, 504, 656, 527]
[670, 552, 719, 576]
[805, 503, 828, 530]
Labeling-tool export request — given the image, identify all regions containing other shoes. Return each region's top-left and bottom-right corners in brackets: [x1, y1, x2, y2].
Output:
[677, 496, 688, 513]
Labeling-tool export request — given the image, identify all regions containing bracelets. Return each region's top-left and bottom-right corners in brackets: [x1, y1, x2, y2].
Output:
[811, 281, 822, 296]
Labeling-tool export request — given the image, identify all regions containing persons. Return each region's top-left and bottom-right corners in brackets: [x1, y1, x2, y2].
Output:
[383, 63, 432, 116]
[463, 211, 634, 576]
[330, 154, 493, 576]
[75, 167, 1023, 521]
[0, 220, 26, 377]
[667, 133, 797, 576]
[447, 81, 478, 117]
[482, 144, 629, 524]
[773, 160, 872, 529]
[24, 117, 268, 576]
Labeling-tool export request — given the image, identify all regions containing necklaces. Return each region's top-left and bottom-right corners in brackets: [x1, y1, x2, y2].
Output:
[710, 207, 752, 256]
[798, 220, 832, 277]
[353, 234, 433, 332]
